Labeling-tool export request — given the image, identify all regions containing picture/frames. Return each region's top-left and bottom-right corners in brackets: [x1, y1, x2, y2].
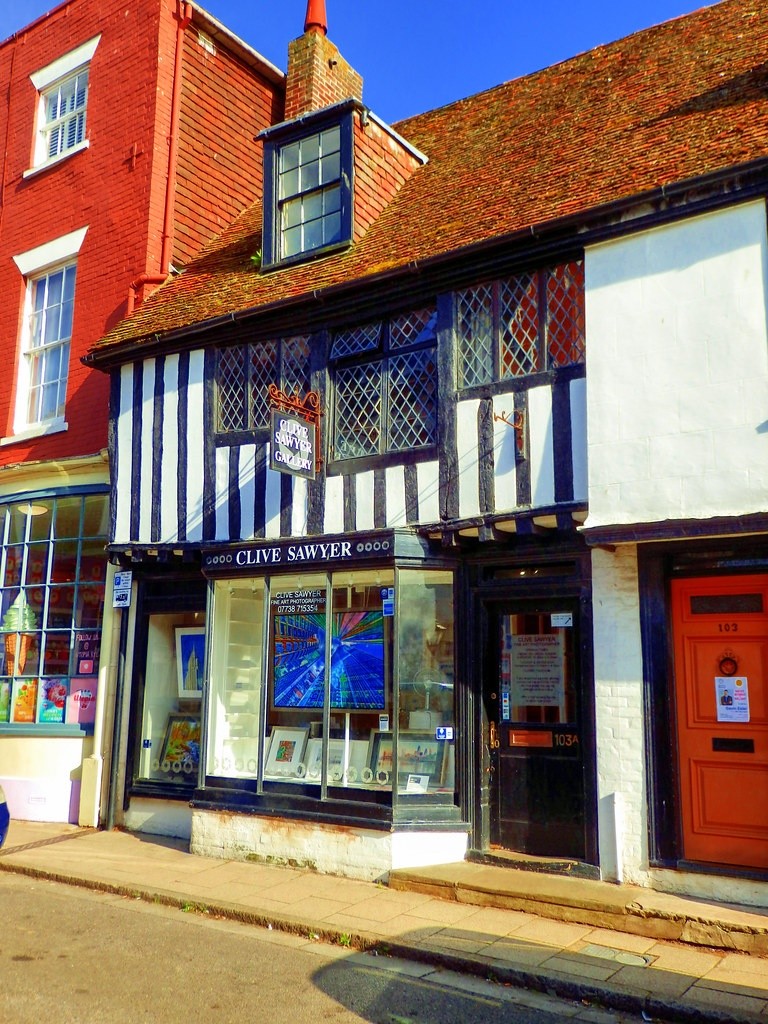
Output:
[364, 728, 449, 788]
[264, 726, 310, 777]
[173, 623, 206, 700]
[306, 741, 352, 777]
[159, 715, 201, 767]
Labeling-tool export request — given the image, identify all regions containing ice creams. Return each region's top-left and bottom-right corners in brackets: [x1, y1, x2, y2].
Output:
[3, 591, 38, 676]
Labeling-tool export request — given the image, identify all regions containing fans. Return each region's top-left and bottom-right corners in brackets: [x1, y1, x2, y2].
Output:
[413, 667, 443, 713]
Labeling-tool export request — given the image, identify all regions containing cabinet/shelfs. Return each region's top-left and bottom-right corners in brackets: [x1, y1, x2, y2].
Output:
[26, 607, 73, 665]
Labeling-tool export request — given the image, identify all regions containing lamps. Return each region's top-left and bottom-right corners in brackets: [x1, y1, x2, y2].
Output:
[17, 505, 48, 516]
